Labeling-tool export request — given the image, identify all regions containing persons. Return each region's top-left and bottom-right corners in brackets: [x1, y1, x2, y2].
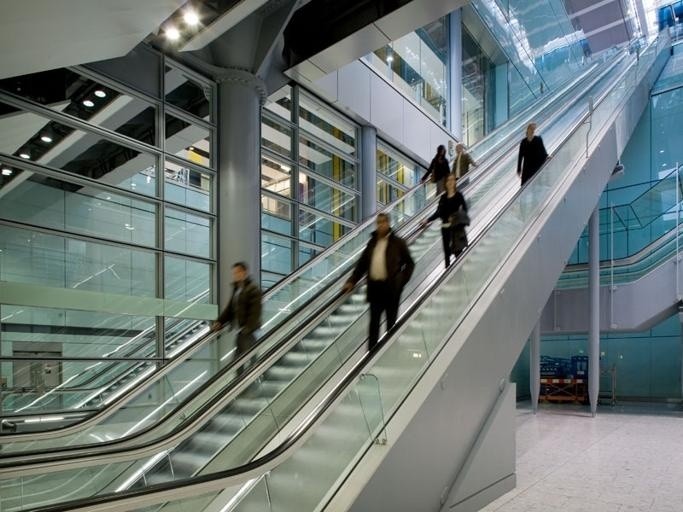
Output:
[451, 142, 479, 183]
[211, 260, 263, 377]
[420, 144, 451, 197]
[335, 211, 417, 354]
[419, 174, 470, 268]
[514, 121, 553, 220]
